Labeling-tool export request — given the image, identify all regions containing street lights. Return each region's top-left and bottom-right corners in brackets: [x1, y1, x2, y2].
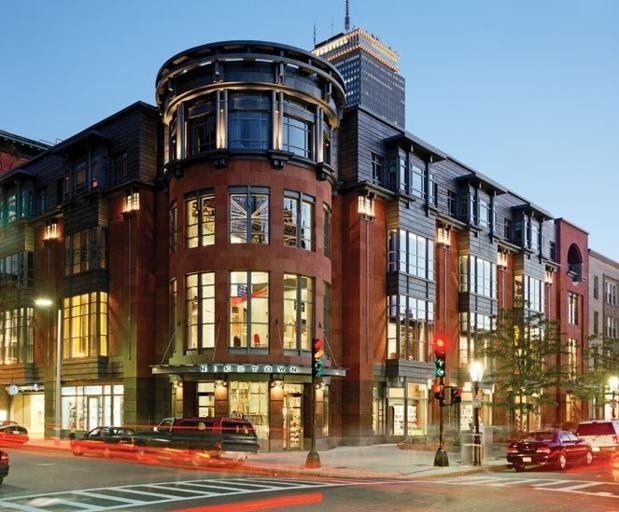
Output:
[608, 376, 619, 419]
[33, 298, 64, 444]
[468, 361, 486, 466]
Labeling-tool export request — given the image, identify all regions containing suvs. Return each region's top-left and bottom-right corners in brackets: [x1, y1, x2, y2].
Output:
[575, 418, 619, 459]
[130, 415, 260, 467]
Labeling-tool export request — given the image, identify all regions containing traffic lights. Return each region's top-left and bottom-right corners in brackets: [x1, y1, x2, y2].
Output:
[311, 337, 324, 380]
[451, 386, 461, 403]
[433, 334, 449, 354]
[433, 384, 445, 400]
[434, 354, 446, 378]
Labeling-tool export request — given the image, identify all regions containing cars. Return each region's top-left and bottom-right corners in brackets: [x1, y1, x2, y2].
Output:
[69, 424, 135, 459]
[0, 420, 30, 444]
[506, 429, 593, 472]
[608, 450, 619, 481]
[0, 448, 9, 485]
